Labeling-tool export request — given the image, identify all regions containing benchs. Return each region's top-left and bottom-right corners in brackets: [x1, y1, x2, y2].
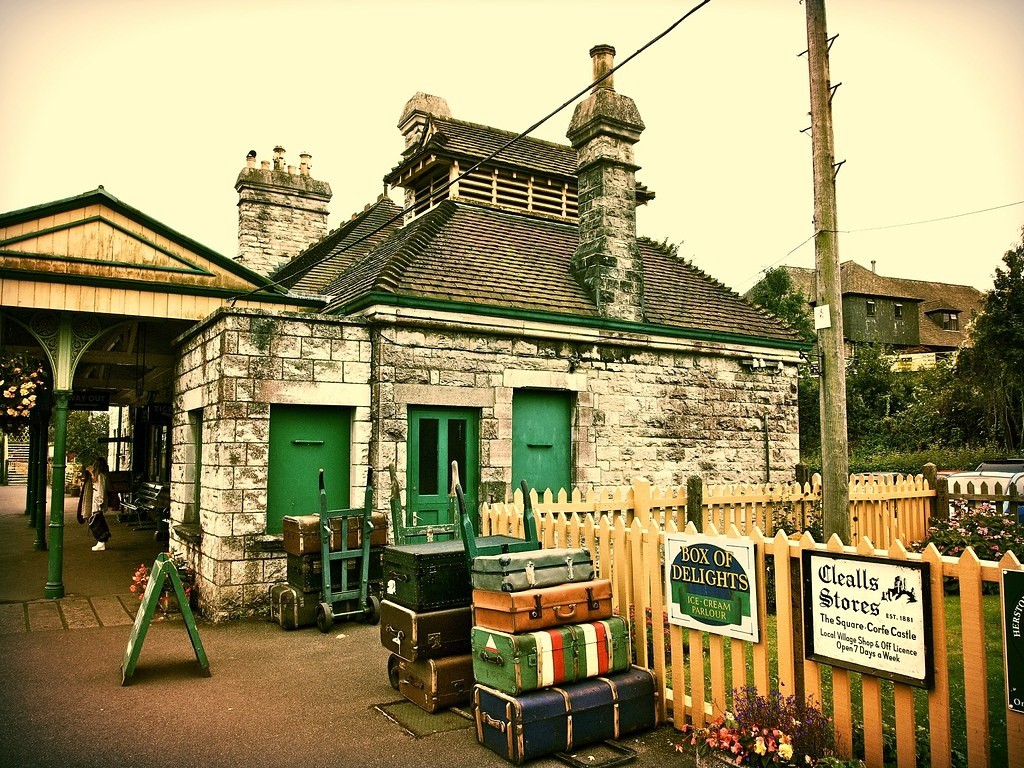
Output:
[118, 481, 163, 529]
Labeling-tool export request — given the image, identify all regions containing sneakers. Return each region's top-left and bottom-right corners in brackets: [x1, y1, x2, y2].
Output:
[92, 542, 105, 551]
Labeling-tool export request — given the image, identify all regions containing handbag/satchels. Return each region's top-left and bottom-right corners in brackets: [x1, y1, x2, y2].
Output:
[88, 510, 111, 542]
[106, 477, 120, 511]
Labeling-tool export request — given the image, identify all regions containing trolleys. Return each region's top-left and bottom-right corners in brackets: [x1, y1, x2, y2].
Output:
[313, 464, 641, 767]
[388, 461, 462, 546]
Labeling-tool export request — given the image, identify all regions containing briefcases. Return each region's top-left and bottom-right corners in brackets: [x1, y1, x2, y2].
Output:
[471, 580, 613, 634]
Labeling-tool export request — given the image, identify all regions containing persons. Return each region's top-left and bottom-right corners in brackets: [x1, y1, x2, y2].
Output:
[85, 456, 111, 551]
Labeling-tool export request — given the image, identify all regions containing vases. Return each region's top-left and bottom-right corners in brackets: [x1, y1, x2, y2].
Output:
[695, 743, 752, 768]
[157, 586, 190, 611]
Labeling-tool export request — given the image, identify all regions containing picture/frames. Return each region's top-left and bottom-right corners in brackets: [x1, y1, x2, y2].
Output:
[663, 532, 760, 644]
[801, 548, 935, 688]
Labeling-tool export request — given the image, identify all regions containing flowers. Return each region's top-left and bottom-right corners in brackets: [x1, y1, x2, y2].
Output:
[674, 681, 867, 768]
[129, 547, 194, 600]
